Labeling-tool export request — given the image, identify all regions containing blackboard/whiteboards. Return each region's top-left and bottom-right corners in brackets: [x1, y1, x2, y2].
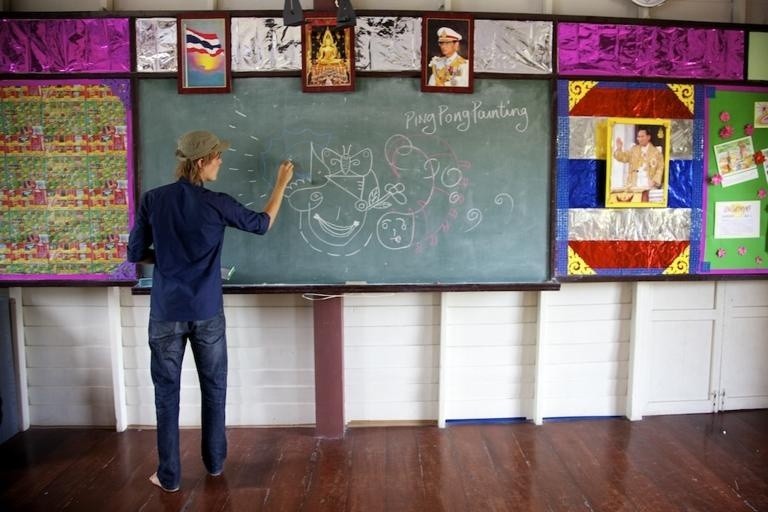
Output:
[129, 70, 561, 293]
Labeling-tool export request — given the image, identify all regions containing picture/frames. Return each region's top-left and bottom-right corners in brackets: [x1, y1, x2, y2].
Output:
[176, 13, 474, 93]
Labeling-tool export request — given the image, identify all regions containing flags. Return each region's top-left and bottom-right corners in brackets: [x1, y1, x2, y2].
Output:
[185, 27, 224, 55]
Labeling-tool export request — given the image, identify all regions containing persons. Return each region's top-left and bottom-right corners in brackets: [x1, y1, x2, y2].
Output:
[613, 126, 667, 202]
[429, 25, 470, 92]
[121, 126, 295, 492]
[311, 30, 346, 64]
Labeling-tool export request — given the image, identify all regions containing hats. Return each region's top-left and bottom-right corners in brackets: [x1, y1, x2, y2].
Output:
[437, 27, 462, 43]
[176, 132, 230, 161]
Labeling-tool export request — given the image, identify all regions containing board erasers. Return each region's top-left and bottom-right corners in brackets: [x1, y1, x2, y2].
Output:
[345, 281, 367, 285]
[139, 277, 154, 286]
